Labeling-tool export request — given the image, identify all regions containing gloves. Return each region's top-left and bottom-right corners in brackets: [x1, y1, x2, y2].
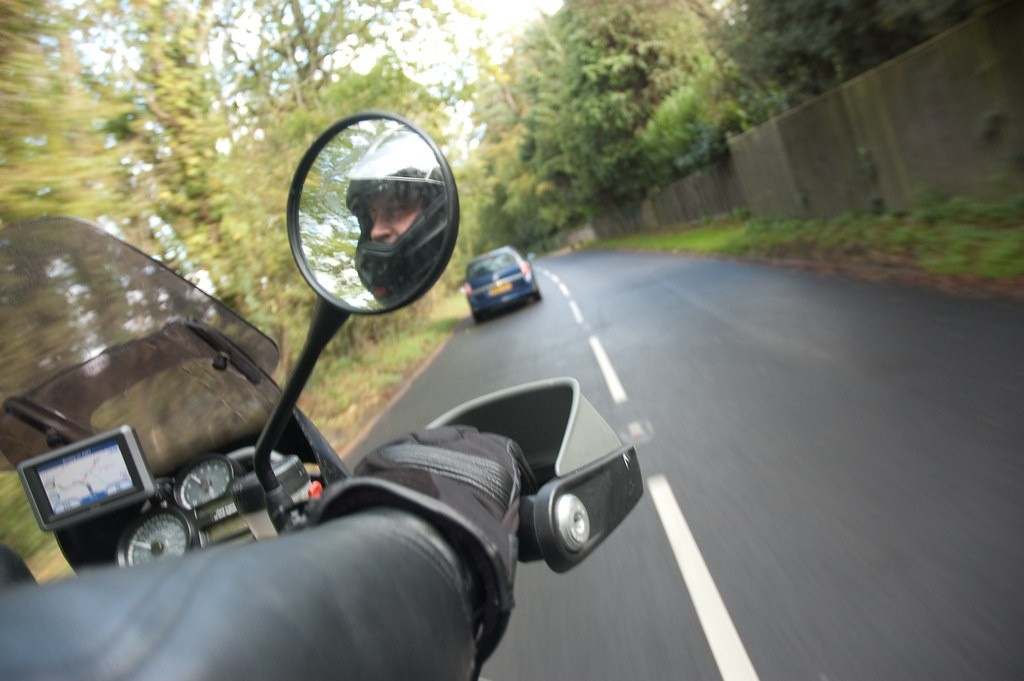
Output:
[317, 423, 541, 681]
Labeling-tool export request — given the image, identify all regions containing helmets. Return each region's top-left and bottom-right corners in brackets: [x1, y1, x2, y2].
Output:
[345, 126, 447, 309]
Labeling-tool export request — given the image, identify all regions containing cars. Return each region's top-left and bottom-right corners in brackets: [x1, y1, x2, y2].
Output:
[462, 245, 543, 322]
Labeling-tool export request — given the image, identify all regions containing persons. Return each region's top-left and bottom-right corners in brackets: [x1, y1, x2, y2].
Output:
[345, 125, 448, 305]
[1, 425, 537, 681]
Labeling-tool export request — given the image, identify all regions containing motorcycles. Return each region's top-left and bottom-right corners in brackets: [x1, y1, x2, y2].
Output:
[0, 114, 646, 681]
